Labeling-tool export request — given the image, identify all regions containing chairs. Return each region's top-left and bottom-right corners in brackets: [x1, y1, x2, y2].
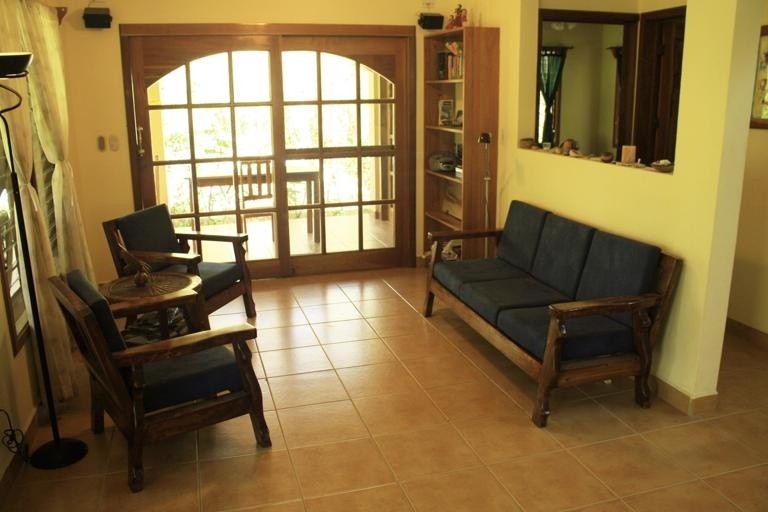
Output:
[102, 203, 256, 330]
[239, 161, 274, 251]
[49, 269, 273, 494]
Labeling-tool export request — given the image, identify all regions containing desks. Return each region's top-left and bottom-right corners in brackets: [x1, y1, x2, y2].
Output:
[184, 168, 321, 252]
[99, 271, 211, 332]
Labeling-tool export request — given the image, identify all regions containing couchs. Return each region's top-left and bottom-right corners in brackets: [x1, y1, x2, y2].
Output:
[424, 200, 683, 428]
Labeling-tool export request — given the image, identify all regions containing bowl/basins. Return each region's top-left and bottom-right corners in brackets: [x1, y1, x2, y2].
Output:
[650, 163, 673, 172]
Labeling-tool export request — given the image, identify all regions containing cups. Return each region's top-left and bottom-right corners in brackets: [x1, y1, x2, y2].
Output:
[543, 143, 551, 151]
[622, 145, 636, 164]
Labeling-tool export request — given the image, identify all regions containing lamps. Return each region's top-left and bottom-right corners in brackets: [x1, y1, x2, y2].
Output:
[0, 52, 88, 470]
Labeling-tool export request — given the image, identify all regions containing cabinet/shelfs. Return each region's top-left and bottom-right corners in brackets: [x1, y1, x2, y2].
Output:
[422, 26, 500, 266]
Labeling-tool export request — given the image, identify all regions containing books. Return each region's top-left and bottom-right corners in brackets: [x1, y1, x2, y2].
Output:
[437, 98, 455, 126]
[435, 36, 464, 80]
[454, 141, 463, 173]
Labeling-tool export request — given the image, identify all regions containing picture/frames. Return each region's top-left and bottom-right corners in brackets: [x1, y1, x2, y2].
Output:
[749, 24, 768, 130]
